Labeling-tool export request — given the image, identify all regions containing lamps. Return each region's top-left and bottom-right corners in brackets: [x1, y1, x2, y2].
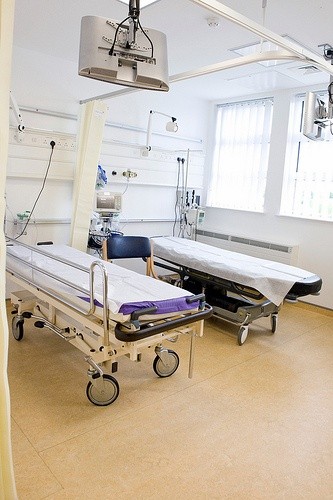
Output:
[143, 110, 178, 157]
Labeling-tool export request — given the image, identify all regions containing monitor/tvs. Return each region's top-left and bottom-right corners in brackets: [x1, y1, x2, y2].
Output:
[96, 192, 121, 210]
[77, 15, 169, 91]
[303, 92, 327, 141]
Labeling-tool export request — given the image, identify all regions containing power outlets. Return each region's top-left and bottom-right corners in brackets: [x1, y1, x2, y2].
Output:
[41, 137, 77, 150]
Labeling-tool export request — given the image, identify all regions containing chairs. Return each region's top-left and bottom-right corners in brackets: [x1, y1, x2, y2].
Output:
[102, 235, 159, 280]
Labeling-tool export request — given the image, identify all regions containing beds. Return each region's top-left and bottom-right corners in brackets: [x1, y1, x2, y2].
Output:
[5, 240, 214, 408]
[143, 235, 322, 345]
